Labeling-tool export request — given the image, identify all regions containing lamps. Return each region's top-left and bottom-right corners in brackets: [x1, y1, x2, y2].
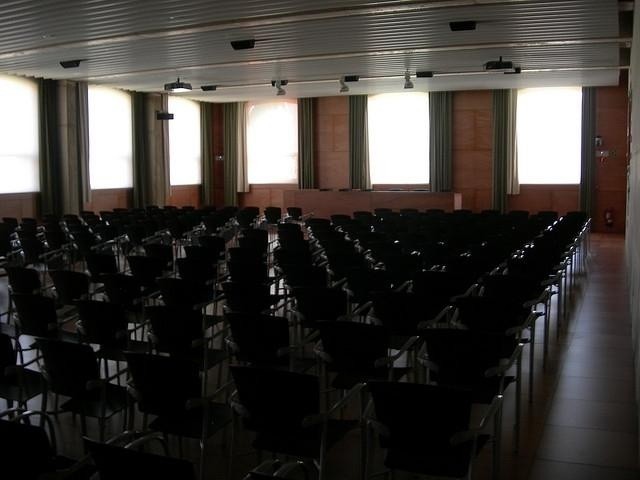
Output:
[337, 77, 350, 93]
[274, 81, 287, 96]
[402, 76, 413, 90]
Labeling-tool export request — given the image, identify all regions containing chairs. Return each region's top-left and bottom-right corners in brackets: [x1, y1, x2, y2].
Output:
[1, 204, 592, 479]
